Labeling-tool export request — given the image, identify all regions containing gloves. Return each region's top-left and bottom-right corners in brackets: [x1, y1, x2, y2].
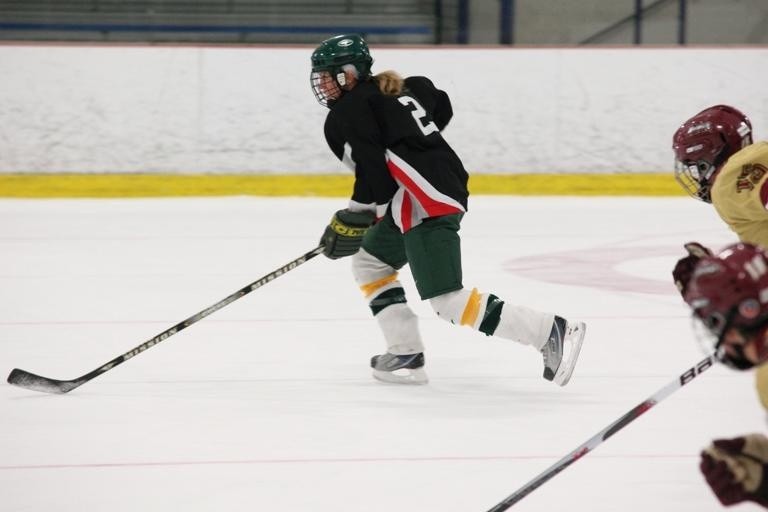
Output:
[699, 430, 768, 511]
[317, 207, 378, 261]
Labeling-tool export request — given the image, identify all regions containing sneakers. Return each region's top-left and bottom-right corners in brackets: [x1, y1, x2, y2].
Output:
[539, 314, 569, 387]
[368, 350, 426, 372]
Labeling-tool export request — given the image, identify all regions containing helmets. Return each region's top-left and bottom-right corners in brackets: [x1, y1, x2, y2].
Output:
[673, 241, 768, 373]
[671, 104, 753, 205]
[309, 33, 374, 108]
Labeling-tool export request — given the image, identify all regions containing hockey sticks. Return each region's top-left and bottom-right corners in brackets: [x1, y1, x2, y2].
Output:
[6, 215, 385, 395]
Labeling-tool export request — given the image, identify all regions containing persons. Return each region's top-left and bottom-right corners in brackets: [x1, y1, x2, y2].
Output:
[308, 32, 568, 384]
[672, 103, 768, 261]
[671, 237, 768, 512]
